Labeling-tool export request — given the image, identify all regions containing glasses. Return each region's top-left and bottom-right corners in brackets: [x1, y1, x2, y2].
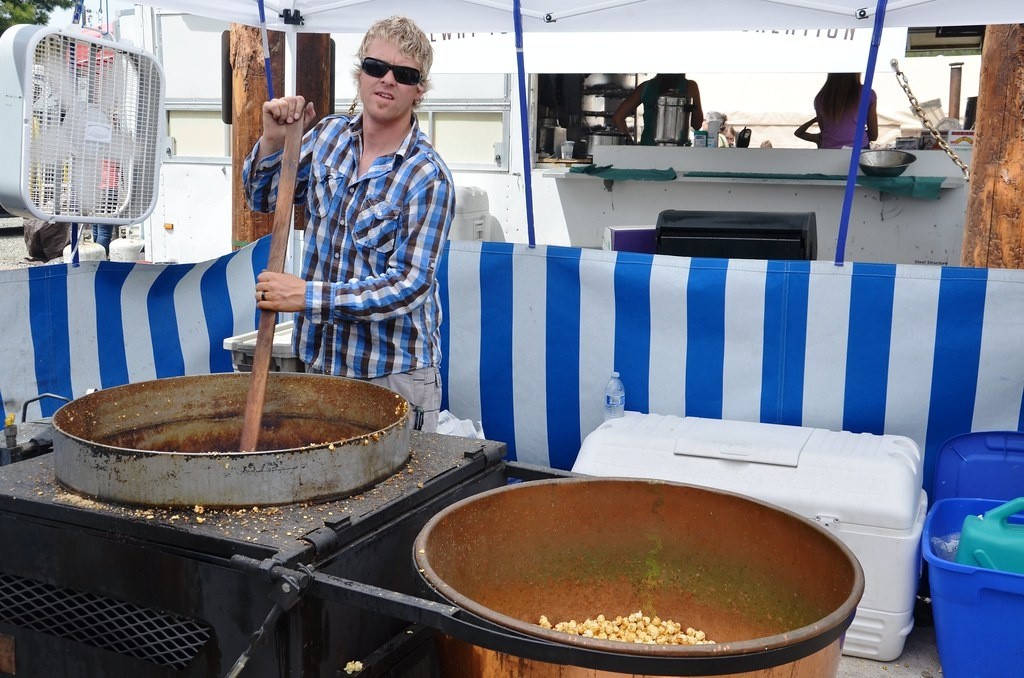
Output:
[360, 57, 422, 85]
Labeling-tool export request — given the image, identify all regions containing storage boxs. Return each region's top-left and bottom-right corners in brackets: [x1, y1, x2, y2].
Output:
[894, 130, 975, 150]
[571, 409, 928, 660]
[922, 496, 1024, 677]
[445, 186, 490, 242]
[608, 226, 655, 254]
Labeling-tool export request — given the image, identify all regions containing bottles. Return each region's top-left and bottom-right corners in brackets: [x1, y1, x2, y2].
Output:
[603, 372, 624, 422]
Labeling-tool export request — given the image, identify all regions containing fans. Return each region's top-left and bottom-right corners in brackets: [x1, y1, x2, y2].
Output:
[0, 23, 166, 226]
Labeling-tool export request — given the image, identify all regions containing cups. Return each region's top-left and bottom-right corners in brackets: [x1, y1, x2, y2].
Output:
[561, 141, 574, 160]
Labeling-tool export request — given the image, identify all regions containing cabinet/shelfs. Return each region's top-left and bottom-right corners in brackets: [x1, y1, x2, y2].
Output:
[576, 73, 639, 145]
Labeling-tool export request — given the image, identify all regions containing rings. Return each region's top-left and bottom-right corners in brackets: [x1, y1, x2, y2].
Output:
[262, 291, 266, 300]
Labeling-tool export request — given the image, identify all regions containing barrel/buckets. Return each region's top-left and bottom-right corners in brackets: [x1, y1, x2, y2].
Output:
[415, 477, 865, 678]
[51, 371, 412, 507]
[922, 498, 1024, 678]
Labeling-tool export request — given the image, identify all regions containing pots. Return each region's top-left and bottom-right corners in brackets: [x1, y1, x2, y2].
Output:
[581, 127, 627, 159]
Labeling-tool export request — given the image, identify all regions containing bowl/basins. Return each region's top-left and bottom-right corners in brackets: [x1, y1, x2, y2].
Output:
[859, 150, 917, 177]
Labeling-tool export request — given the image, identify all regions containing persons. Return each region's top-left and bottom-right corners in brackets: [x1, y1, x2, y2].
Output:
[794, 73, 878, 149]
[685, 111, 736, 147]
[614, 73, 703, 146]
[760, 141, 772, 148]
[243, 16, 456, 432]
[92, 159, 121, 256]
